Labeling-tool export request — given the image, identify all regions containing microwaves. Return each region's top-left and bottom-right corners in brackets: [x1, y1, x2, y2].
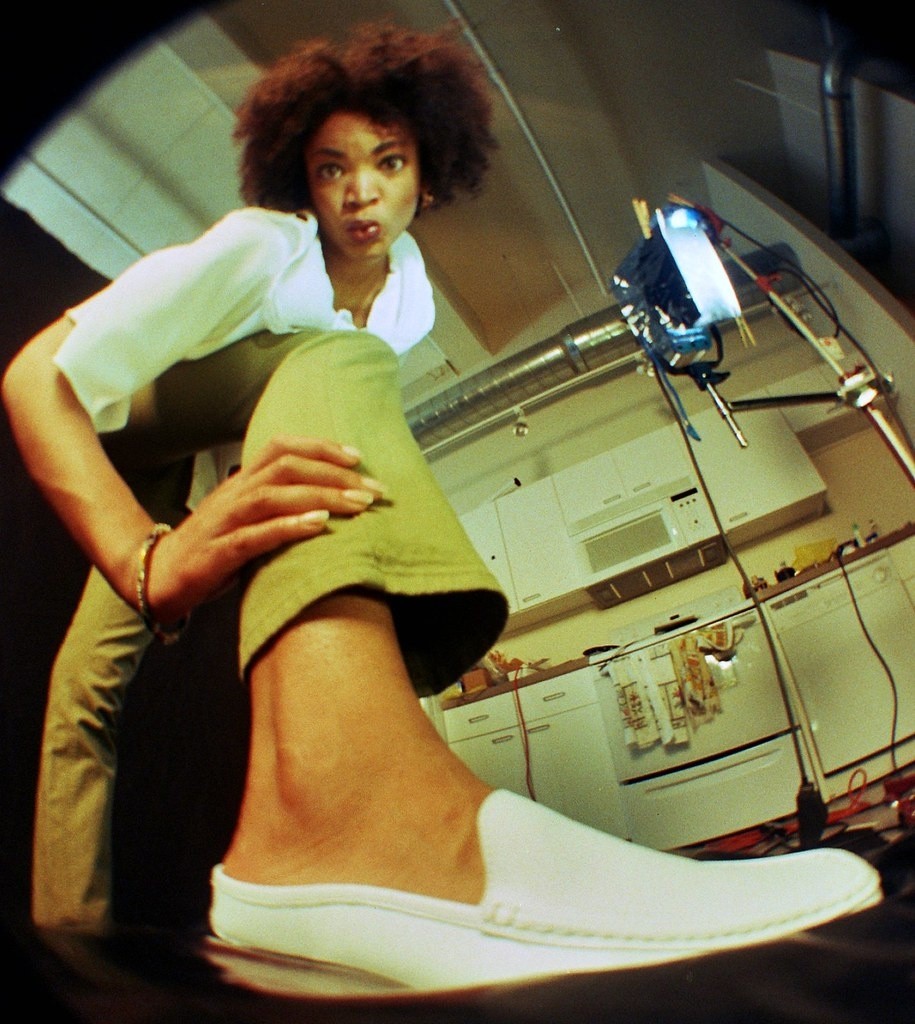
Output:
[569, 484, 724, 610]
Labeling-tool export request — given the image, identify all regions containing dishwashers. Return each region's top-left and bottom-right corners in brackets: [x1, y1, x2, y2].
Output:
[589, 601, 808, 852]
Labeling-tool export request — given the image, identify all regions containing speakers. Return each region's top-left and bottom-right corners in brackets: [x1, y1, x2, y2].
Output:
[612, 205, 741, 357]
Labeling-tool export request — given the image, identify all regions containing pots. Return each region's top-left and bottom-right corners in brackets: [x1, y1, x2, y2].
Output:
[654, 614, 698, 633]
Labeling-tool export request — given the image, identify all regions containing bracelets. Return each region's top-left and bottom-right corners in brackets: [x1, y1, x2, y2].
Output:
[136, 523, 192, 645]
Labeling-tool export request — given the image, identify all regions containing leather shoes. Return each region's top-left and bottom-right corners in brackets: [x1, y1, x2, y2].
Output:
[203, 789, 882, 999]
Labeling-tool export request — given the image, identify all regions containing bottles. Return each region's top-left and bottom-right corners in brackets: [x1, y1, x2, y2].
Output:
[850, 522, 868, 548]
[867, 519, 883, 538]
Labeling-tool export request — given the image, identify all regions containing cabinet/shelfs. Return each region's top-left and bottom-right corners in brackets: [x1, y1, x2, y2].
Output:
[755, 524, 915, 806]
[456, 477, 592, 638]
[549, 425, 697, 537]
[767, 356, 900, 455]
[439, 658, 631, 844]
[673, 390, 827, 547]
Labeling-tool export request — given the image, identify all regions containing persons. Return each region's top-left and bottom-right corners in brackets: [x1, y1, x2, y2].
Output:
[0, 19, 885, 1001]
